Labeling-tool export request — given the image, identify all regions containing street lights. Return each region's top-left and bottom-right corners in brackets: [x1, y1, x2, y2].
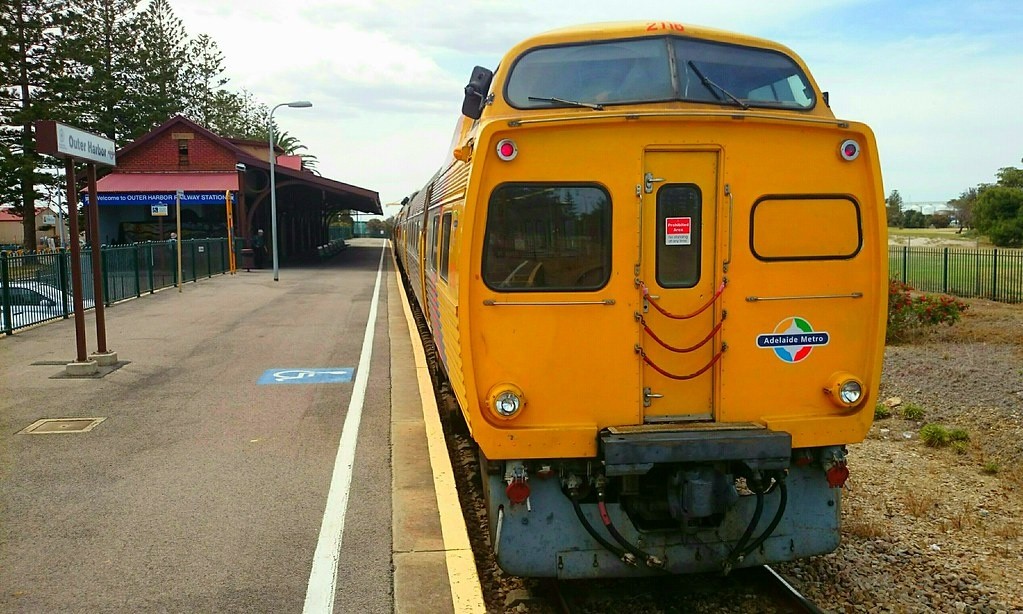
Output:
[270, 101, 313, 278]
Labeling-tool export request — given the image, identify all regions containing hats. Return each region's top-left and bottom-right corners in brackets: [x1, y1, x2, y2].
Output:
[170, 232, 177, 239]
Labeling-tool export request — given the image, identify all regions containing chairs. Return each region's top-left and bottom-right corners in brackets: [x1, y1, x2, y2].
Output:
[315, 237, 350, 261]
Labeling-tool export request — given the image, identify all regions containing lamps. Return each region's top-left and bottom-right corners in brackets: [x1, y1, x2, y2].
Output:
[235, 162, 246, 171]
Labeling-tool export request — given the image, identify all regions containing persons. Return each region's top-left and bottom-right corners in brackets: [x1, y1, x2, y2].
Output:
[249, 229, 268, 269]
[170, 233, 178, 242]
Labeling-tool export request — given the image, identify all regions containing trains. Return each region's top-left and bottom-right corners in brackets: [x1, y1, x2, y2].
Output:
[391, 23, 889, 582]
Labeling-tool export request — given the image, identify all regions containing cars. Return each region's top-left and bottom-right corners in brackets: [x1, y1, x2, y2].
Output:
[0, 308, 56, 332]
[0, 282, 95, 316]
